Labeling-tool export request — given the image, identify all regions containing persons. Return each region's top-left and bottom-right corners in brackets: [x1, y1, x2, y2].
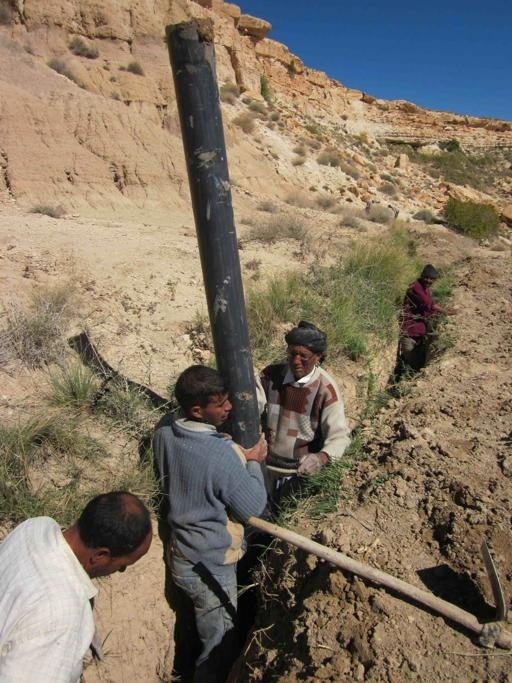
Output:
[396, 263, 456, 364]
[0, 490, 152, 682]
[255, 319, 350, 524]
[153, 363, 270, 681]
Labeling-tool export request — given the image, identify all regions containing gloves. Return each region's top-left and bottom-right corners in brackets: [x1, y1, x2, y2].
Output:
[297, 453, 322, 477]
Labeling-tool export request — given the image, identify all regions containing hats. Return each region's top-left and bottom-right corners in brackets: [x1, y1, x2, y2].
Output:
[420, 264, 438, 279]
[284, 320, 328, 352]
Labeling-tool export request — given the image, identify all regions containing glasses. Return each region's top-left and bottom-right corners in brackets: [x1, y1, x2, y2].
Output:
[286, 347, 316, 361]
